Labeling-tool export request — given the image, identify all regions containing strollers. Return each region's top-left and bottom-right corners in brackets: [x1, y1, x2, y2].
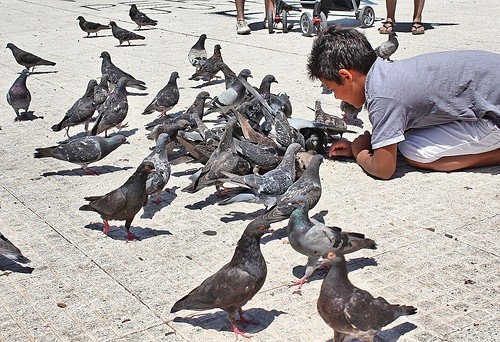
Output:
[267, 0, 376, 37]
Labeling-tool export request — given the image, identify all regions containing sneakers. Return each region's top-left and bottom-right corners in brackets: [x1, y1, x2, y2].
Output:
[236, 19, 251, 34]
[264, 18, 276, 28]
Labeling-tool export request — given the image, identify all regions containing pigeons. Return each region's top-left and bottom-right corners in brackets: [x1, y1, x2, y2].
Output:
[99, 51, 149, 91]
[315, 246, 418, 342]
[0, 230, 33, 267]
[255, 152, 326, 224]
[286, 194, 378, 289]
[187, 44, 223, 85]
[128, 3, 159, 31]
[374, 30, 400, 63]
[33, 135, 131, 176]
[340, 101, 363, 122]
[107, 20, 145, 47]
[77, 159, 157, 242]
[218, 142, 305, 207]
[88, 76, 130, 138]
[299, 127, 329, 153]
[6, 68, 32, 123]
[314, 99, 347, 139]
[50, 79, 99, 138]
[76, 15, 111, 39]
[168, 218, 276, 341]
[141, 69, 307, 199]
[269, 0, 303, 16]
[188, 33, 208, 72]
[6, 42, 57, 74]
[214, 62, 237, 90]
[93, 73, 112, 111]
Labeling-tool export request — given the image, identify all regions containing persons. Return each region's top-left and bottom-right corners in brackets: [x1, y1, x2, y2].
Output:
[306, 28, 500, 179]
[379, 0, 425, 34]
[235, 0, 282, 33]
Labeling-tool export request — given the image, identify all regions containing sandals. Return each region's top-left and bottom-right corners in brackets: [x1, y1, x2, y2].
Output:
[379, 20, 395, 34]
[412, 21, 424, 34]
